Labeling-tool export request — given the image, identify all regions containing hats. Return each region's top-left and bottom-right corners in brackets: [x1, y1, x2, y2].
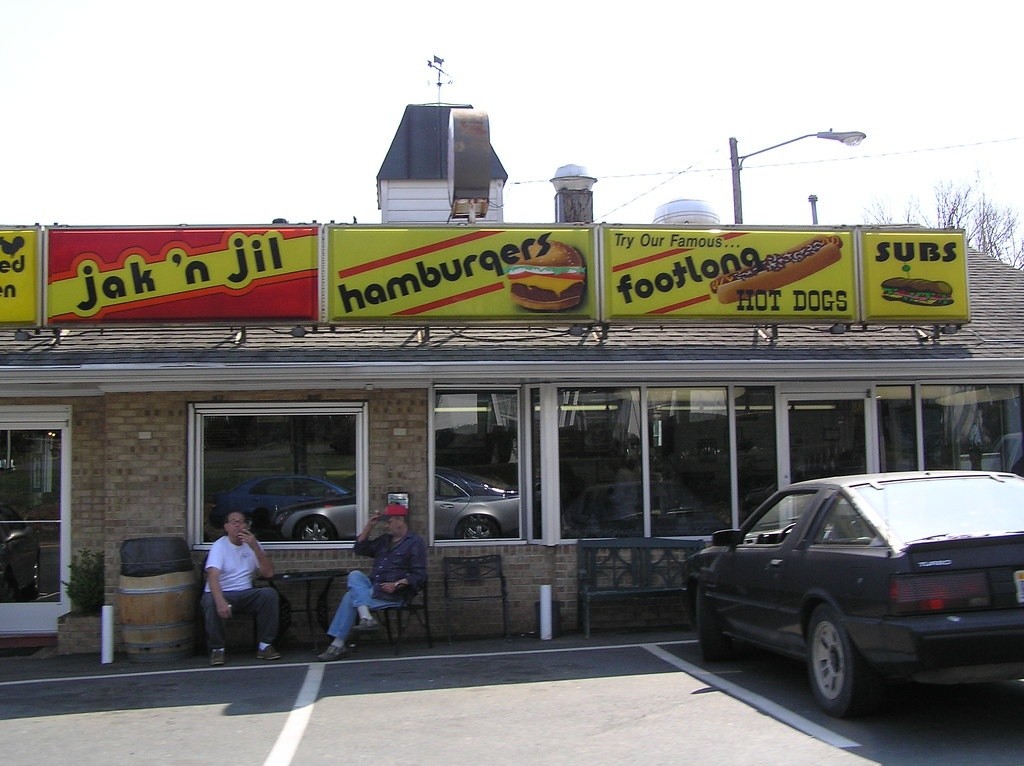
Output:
[371, 504, 408, 520]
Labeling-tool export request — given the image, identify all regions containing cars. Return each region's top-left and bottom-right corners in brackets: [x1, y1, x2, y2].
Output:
[212, 472, 351, 534]
[563, 478, 730, 540]
[272, 465, 520, 541]
[0, 504, 42, 603]
[682, 469, 1024, 717]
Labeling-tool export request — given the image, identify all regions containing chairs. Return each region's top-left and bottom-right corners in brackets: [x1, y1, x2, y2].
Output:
[442, 554, 508, 643]
[361, 573, 434, 653]
[200, 555, 258, 651]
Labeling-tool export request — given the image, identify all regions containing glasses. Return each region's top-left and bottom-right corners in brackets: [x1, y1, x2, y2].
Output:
[228, 519, 248, 525]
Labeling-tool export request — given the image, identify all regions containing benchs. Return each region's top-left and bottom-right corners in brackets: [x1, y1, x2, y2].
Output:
[577, 538, 706, 640]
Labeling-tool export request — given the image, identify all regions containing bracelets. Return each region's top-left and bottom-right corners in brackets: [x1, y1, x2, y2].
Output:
[394, 581, 400, 589]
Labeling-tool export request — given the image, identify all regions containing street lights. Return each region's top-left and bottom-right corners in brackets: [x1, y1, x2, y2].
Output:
[729, 129, 867, 226]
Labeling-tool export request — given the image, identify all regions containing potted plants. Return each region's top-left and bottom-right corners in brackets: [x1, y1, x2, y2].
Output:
[57, 548, 105, 655]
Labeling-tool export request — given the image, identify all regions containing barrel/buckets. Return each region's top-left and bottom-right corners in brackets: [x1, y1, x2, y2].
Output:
[117, 537, 197, 663]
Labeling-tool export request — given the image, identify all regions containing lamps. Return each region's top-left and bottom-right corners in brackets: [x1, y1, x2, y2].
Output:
[942, 327, 957, 334]
[569, 326, 584, 337]
[291, 326, 307, 338]
[14, 331, 31, 342]
[830, 324, 846, 334]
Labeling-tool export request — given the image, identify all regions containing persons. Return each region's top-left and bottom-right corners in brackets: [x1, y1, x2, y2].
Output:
[199, 509, 281, 665]
[316, 501, 428, 662]
[564, 465, 641, 520]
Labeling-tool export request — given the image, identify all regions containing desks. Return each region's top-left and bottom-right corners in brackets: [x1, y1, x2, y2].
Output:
[258, 567, 347, 655]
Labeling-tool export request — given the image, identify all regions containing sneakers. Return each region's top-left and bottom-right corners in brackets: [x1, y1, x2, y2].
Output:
[318, 644, 350, 661]
[353, 618, 383, 633]
[256, 645, 282, 659]
[210, 648, 225, 665]
